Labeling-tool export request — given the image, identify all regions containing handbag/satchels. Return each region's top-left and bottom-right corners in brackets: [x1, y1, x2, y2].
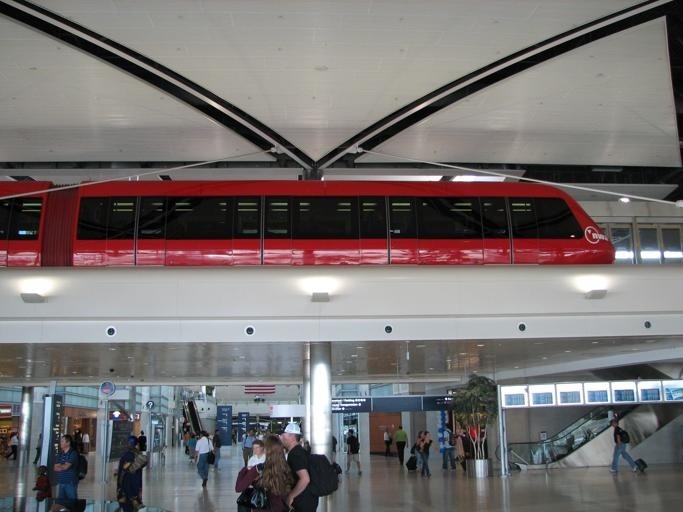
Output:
[208, 451, 215, 465]
[236, 487, 266, 508]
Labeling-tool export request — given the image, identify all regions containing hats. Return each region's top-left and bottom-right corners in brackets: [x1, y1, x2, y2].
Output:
[284, 422, 301, 435]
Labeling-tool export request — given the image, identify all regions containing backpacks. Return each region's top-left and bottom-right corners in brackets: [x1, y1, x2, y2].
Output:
[619, 428, 629, 443]
[306, 453, 342, 497]
[76, 454, 88, 480]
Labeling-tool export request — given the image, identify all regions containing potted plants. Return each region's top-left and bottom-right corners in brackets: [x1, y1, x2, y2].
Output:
[449, 374, 497, 479]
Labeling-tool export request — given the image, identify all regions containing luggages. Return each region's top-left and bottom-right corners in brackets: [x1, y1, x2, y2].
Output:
[634, 458, 647, 472]
[406, 455, 417, 471]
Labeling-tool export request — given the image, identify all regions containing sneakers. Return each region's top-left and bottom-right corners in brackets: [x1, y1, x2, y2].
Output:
[201, 479, 207, 487]
[423, 472, 432, 480]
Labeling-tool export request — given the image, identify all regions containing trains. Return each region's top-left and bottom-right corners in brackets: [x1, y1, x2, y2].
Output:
[0, 178, 617, 270]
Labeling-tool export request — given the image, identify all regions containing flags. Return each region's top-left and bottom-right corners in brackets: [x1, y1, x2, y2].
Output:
[245, 386, 275, 394]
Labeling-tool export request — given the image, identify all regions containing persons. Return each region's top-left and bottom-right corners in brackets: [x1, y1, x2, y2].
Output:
[116, 429, 148, 512]
[384, 426, 471, 479]
[235, 422, 320, 512]
[6, 432, 19, 460]
[333, 429, 362, 476]
[610, 420, 638, 473]
[32, 428, 89, 498]
[183, 429, 222, 487]
